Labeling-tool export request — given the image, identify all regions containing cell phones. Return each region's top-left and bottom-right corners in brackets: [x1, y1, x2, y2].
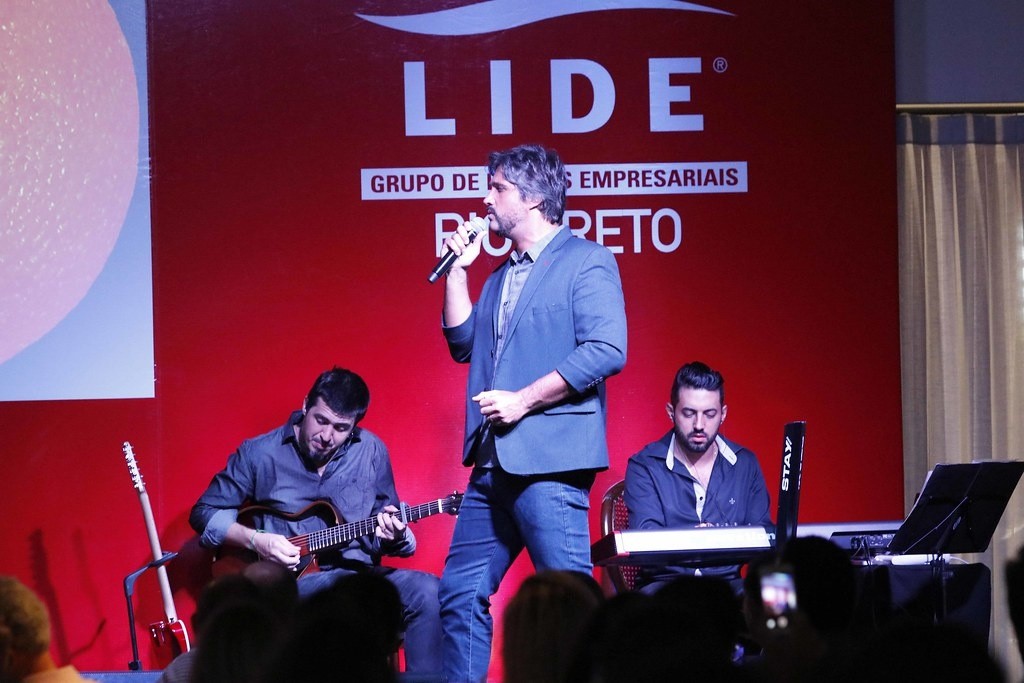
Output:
[757, 563, 799, 636]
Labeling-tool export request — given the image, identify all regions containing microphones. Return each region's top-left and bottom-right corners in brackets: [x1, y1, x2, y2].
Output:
[427, 217, 487, 284]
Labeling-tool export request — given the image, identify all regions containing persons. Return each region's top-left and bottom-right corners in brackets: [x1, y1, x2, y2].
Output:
[189, 369, 447, 683]
[1, 536, 1024, 683]
[438, 145, 627, 682]
[623, 362, 776, 598]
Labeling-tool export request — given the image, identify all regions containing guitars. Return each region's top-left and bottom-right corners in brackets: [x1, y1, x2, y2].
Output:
[211, 490, 464, 585]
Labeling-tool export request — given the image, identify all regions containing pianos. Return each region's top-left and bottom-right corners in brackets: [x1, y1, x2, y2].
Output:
[592, 516, 902, 573]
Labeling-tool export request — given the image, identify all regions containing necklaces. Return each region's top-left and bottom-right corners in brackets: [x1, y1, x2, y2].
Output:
[676, 442, 717, 490]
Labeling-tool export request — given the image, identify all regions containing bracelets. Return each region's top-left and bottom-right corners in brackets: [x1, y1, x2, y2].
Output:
[250, 529, 266, 550]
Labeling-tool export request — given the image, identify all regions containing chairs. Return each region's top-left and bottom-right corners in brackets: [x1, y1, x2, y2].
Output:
[600, 480, 641, 593]
